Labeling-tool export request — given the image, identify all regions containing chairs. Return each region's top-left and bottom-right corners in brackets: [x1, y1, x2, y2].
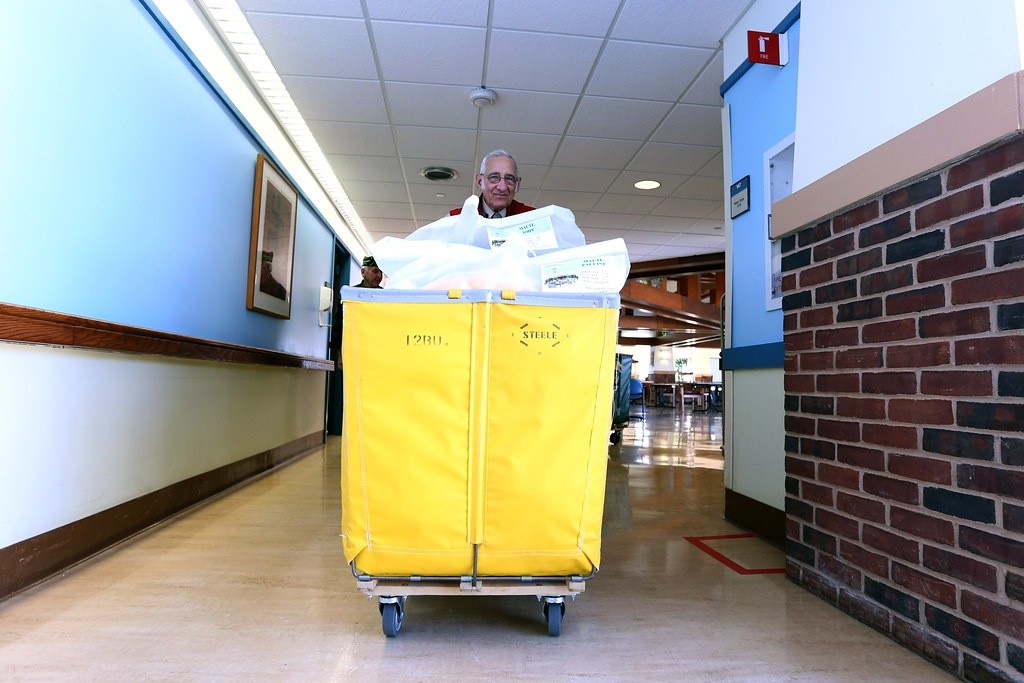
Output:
[629, 379, 644, 421]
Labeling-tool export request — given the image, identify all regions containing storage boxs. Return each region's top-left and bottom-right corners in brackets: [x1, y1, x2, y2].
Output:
[610, 353, 638, 442]
[339, 285, 621, 638]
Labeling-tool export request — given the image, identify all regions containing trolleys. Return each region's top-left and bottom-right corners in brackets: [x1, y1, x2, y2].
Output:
[610, 351, 639, 444]
[339, 281, 622, 638]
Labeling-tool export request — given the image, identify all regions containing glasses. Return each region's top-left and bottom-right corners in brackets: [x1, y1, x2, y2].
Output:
[480, 171, 519, 185]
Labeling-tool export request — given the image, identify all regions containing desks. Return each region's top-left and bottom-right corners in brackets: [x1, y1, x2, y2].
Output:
[693, 383, 722, 411]
[642, 383, 683, 407]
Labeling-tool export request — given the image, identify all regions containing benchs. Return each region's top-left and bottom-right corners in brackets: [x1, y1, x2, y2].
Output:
[663, 394, 702, 406]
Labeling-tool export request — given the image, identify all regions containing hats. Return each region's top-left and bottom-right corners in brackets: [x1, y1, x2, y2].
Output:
[362, 255, 380, 267]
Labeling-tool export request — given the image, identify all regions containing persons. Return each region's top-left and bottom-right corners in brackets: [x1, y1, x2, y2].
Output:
[332, 257, 382, 370]
[259, 250, 289, 303]
[445, 150, 535, 220]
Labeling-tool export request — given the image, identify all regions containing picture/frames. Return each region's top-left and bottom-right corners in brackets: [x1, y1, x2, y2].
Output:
[247, 154, 299, 320]
